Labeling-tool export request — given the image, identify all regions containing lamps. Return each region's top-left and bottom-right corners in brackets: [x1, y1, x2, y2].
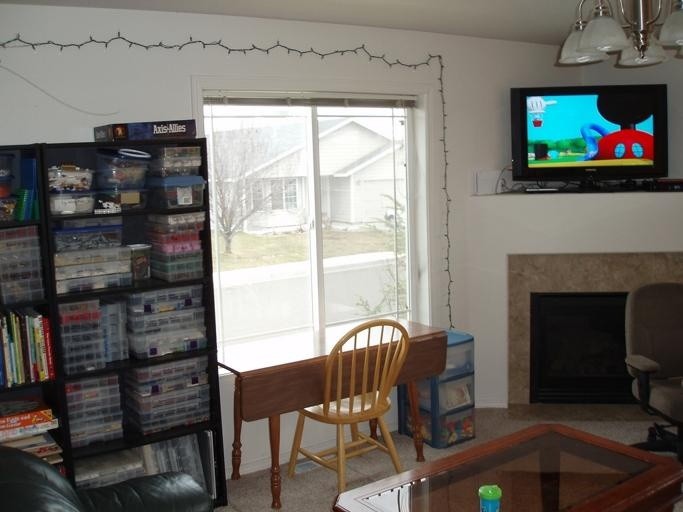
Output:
[552, 0, 683, 72]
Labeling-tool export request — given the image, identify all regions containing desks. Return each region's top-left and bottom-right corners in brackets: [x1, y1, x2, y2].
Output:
[331, 419, 681, 512]
[211, 318, 454, 512]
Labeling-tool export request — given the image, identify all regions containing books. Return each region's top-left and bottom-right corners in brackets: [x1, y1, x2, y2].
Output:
[0, 307, 56, 389]
[0, 398, 64, 466]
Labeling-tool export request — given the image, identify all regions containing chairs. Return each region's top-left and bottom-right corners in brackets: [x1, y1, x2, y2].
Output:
[287, 318, 412, 498]
[620, 279, 683, 464]
[0, 445, 211, 510]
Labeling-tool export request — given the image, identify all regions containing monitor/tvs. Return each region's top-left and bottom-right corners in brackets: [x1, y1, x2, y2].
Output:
[510, 84, 667, 181]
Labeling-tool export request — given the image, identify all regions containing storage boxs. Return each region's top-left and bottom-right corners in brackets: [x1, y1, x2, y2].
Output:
[51, 143, 212, 491]
[0, 225, 45, 305]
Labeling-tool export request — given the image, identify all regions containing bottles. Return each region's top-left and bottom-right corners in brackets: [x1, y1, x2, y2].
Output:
[477, 484, 501, 512]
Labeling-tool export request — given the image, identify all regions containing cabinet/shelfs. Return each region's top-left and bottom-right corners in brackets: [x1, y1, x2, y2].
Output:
[0, 141, 72, 481]
[40, 137, 231, 510]
[396, 327, 477, 447]
